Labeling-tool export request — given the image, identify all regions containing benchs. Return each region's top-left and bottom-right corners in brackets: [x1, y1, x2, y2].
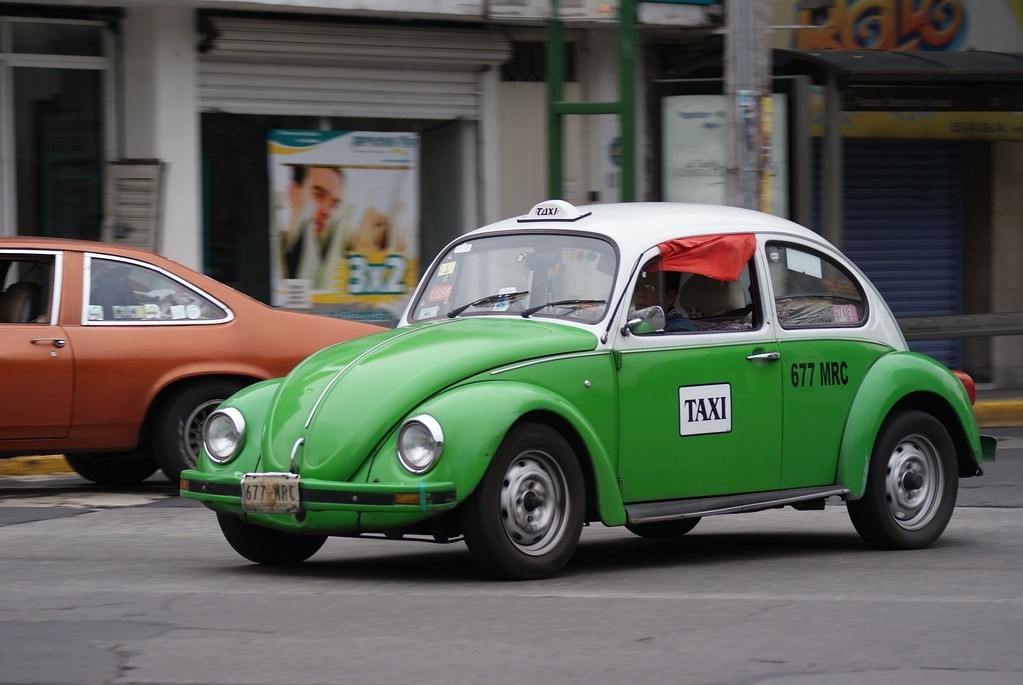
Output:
[748, 299, 837, 327]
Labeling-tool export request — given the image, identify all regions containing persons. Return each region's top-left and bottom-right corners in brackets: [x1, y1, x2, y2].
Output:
[632, 257, 699, 333]
[279, 164, 351, 278]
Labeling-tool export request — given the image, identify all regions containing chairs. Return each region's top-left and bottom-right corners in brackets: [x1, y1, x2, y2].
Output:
[680, 272, 750, 330]
[0, 282, 41, 323]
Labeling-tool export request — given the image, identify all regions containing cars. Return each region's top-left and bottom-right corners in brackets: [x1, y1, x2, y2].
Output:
[175, 198, 999, 586]
[0, 231, 399, 496]
